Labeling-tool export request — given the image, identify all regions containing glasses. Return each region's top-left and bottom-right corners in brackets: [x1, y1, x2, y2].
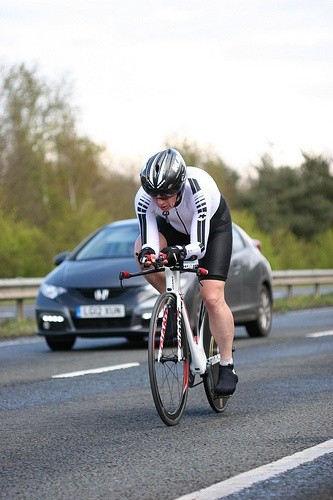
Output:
[151, 190, 176, 199]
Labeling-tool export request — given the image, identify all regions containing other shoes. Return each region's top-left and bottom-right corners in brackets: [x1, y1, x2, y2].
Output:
[214, 364, 238, 395]
[188, 367, 194, 387]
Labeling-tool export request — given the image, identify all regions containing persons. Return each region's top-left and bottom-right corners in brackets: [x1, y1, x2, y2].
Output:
[135, 149, 240, 396]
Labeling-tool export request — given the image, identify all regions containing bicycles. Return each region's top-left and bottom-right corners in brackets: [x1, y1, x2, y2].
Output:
[119, 255, 239, 426]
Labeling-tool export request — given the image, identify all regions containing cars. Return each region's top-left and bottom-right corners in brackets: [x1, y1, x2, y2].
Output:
[36, 215, 274, 351]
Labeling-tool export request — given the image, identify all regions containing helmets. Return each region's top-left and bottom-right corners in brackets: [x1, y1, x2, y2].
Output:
[140, 148, 185, 195]
